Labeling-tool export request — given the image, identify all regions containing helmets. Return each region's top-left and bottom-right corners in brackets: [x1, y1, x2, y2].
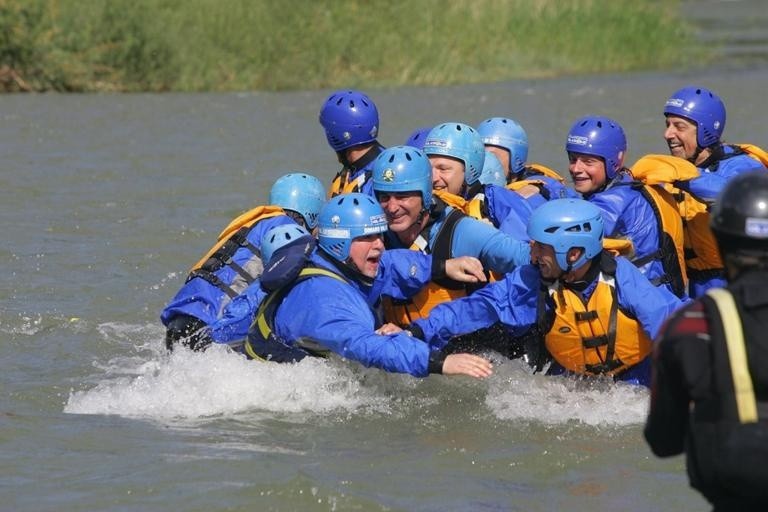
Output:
[708, 169, 766, 256]
[478, 151, 506, 188]
[564, 115, 626, 180]
[476, 117, 529, 173]
[423, 122, 485, 186]
[526, 196, 604, 276]
[371, 144, 432, 210]
[663, 84, 726, 147]
[406, 127, 432, 150]
[270, 172, 325, 230]
[316, 192, 389, 263]
[318, 89, 379, 152]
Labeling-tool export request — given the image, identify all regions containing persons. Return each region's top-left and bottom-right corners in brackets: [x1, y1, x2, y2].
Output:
[643, 168, 767, 512]
[162, 87, 768, 388]
